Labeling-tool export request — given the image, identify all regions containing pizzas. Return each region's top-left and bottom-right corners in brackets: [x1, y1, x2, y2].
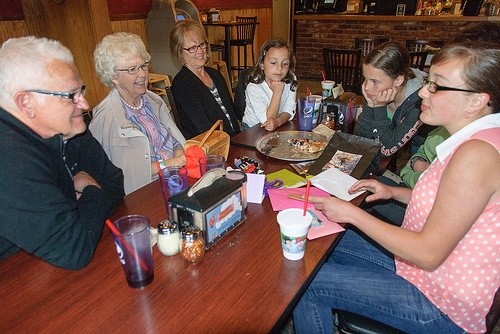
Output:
[287, 138, 326, 153]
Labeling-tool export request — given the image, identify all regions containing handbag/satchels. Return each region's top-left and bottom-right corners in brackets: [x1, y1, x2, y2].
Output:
[306, 132, 381, 180]
[184, 120, 230, 162]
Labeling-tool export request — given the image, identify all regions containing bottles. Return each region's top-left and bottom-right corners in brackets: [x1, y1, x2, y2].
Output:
[179, 226, 206, 262]
[157, 219, 181, 256]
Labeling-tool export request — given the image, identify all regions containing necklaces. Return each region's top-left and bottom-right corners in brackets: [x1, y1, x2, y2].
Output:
[120, 96, 143, 110]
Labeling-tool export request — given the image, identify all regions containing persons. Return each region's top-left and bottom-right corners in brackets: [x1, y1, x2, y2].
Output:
[240, 39, 297, 131]
[364, 124, 451, 225]
[0, 35, 126, 269]
[87, 31, 187, 196]
[354, 40, 439, 173]
[292, 34, 500, 334]
[170, 19, 241, 139]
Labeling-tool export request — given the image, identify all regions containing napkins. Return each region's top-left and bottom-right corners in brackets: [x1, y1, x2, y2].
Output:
[307, 166, 367, 203]
[123, 223, 159, 254]
[311, 123, 336, 140]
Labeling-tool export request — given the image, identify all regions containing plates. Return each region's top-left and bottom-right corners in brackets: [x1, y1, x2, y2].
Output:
[255, 130, 329, 161]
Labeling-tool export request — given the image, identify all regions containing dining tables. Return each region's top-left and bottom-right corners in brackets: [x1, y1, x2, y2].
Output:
[0, 91, 398, 334]
[319, 54, 435, 68]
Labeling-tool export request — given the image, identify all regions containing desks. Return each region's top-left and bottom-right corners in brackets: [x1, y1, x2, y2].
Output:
[202, 20, 261, 91]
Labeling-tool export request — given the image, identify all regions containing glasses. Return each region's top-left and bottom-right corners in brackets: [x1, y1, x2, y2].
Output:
[25, 86, 86, 103]
[183, 42, 208, 54]
[422, 76, 478, 94]
[117, 62, 150, 75]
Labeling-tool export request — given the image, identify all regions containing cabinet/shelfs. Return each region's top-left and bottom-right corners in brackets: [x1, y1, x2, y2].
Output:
[148, 72, 173, 111]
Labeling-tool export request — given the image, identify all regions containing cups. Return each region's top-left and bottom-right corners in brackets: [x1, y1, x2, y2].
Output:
[298, 80, 364, 131]
[276, 207, 312, 261]
[222, 170, 248, 211]
[158, 166, 187, 214]
[110, 214, 154, 288]
[198, 154, 226, 178]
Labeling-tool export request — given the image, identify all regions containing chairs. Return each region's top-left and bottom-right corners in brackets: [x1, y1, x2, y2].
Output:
[405, 39, 430, 52]
[209, 43, 226, 62]
[323, 47, 363, 96]
[224, 15, 258, 81]
[408, 51, 428, 72]
[354, 37, 392, 57]
[166, 85, 184, 135]
